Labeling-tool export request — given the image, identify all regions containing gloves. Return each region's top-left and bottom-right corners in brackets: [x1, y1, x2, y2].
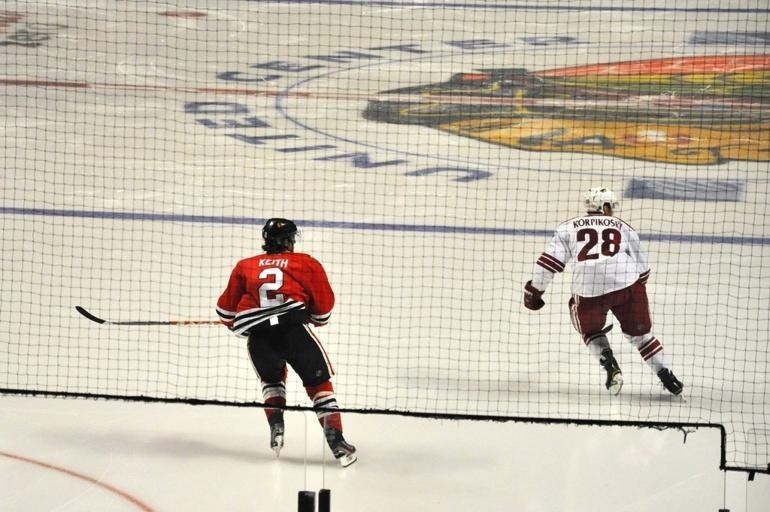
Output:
[523, 280, 545, 311]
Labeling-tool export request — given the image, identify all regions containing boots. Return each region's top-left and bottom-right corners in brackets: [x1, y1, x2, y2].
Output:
[323, 424, 356, 458]
[657, 366, 684, 395]
[269, 409, 284, 448]
[600, 348, 622, 390]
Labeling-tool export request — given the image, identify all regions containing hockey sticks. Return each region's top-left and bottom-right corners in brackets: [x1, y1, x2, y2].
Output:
[74, 304, 224, 326]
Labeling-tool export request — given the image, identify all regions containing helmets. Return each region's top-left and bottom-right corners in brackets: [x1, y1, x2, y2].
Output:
[261, 218, 302, 249]
[583, 186, 620, 215]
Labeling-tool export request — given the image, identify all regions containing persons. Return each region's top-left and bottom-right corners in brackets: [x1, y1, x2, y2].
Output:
[215, 216, 358, 460]
[522, 185, 686, 398]
